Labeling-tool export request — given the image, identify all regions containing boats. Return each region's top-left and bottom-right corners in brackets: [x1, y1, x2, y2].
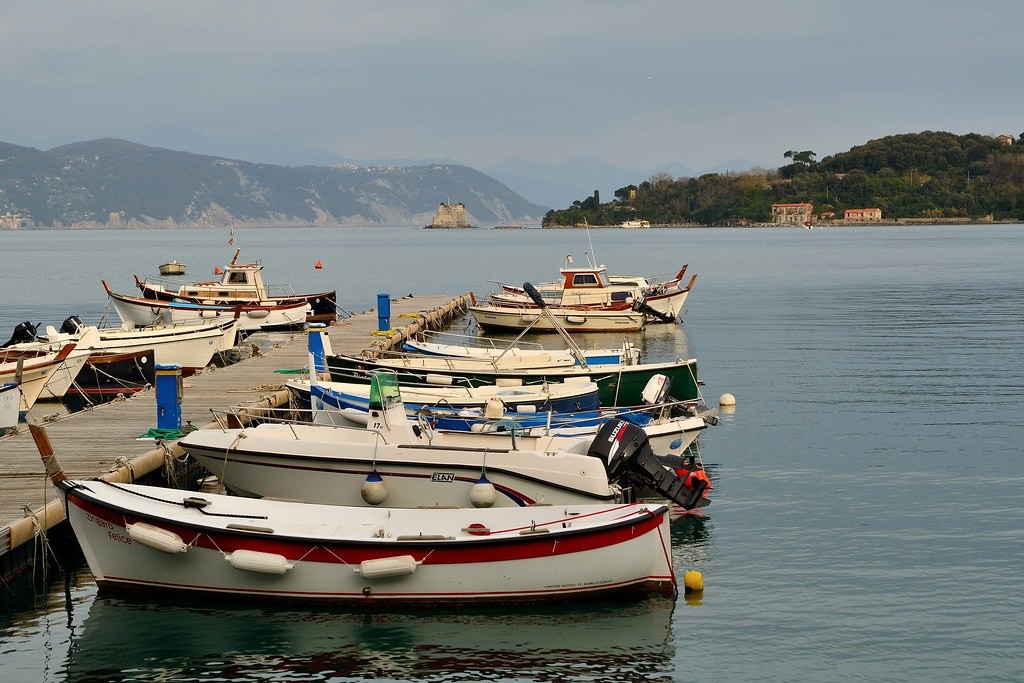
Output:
[0, 215, 723, 610]
